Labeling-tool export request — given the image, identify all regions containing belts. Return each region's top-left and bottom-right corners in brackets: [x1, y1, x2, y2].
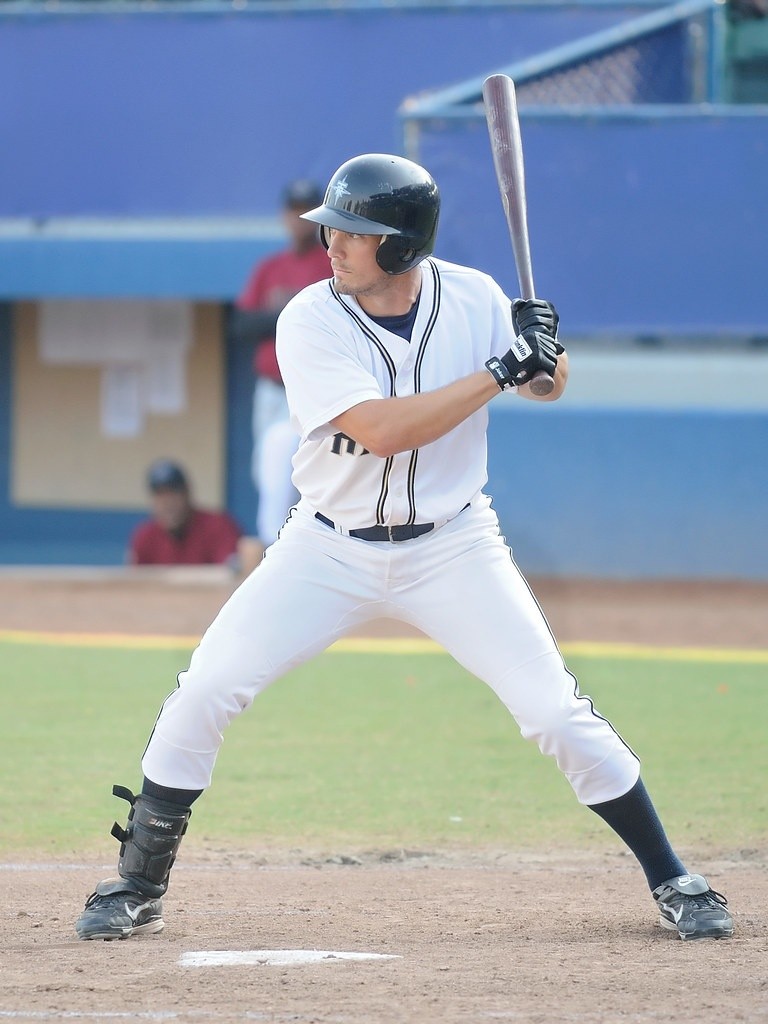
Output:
[314, 502, 471, 541]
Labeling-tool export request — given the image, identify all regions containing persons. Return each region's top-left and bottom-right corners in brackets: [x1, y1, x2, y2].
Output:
[232, 185, 336, 546]
[75, 153, 731, 940]
[120, 459, 241, 565]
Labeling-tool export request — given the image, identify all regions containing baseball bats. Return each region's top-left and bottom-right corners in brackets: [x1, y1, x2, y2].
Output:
[481, 73, 556, 397]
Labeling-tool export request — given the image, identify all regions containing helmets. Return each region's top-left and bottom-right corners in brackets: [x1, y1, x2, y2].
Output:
[298, 153, 440, 275]
[148, 463, 186, 489]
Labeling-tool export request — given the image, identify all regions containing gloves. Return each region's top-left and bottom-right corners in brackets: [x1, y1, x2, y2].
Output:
[483, 330, 565, 394]
[511, 298, 560, 343]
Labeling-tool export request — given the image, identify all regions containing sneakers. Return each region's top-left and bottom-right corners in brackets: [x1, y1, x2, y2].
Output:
[651, 874, 734, 940]
[76, 876, 165, 939]
